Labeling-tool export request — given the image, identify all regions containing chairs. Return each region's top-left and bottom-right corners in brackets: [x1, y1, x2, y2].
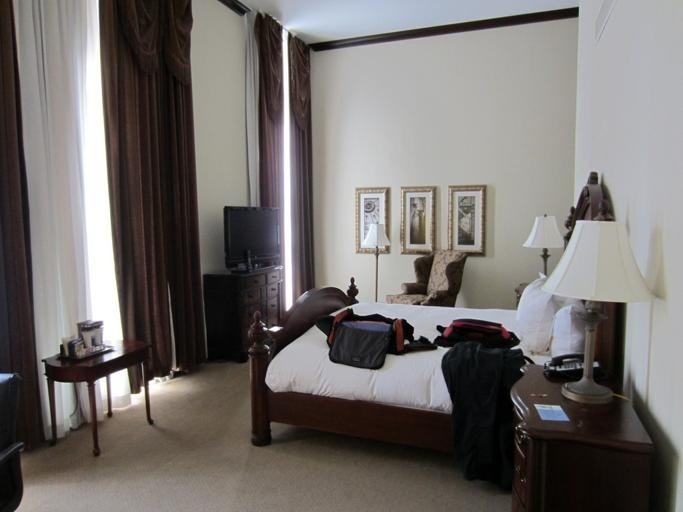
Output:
[385, 251, 467, 307]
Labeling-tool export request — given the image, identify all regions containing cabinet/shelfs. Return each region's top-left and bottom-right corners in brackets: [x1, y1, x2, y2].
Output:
[243, 269, 280, 356]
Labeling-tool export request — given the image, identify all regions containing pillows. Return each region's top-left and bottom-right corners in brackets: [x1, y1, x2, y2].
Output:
[550, 301, 586, 357]
[515, 271, 554, 356]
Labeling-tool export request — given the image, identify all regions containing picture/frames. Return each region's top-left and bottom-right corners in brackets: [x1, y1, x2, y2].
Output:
[401, 186, 434, 255]
[448, 185, 485, 256]
[356, 187, 389, 254]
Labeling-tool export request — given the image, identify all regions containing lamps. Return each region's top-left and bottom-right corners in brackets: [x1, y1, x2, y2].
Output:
[522, 214, 562, 275]
[542, 219, 655, 405]
[362, 222, 391, 301]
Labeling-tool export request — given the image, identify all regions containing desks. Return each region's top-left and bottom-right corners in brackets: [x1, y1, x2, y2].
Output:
[41, 340, 154, 457]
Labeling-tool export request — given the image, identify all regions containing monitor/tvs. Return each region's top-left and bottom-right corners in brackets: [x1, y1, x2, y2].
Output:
[223, 206, 280, 273]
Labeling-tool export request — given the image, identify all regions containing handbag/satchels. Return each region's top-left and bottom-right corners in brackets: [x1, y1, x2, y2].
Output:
[441, 319, 509, 349]
[326, 308, 413, 369]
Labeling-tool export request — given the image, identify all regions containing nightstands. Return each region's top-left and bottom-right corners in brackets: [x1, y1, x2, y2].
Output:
[509, 363, 654, 512]
[515, 282, 527, 308]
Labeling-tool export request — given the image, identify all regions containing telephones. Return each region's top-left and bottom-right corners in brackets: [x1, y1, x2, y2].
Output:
[542, 352, 608, 382]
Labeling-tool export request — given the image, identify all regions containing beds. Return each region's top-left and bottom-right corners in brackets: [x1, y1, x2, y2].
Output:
[247, 168, 617, 447]
